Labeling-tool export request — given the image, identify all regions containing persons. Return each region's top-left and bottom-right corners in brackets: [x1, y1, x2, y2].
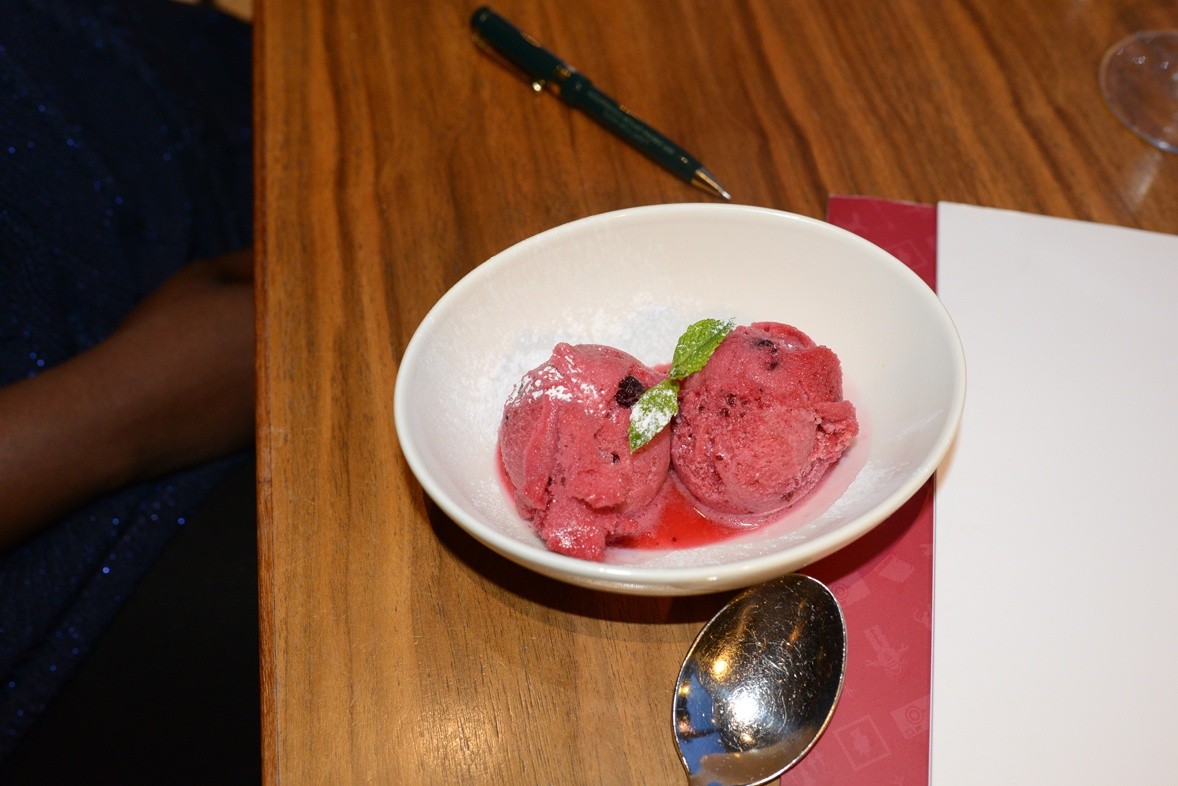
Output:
[0, 1, 263, 786]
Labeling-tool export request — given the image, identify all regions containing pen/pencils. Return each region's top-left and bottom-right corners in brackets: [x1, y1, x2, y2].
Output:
[469, 6, 732, 202]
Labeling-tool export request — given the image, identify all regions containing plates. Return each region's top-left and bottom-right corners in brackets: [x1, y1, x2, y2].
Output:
[392, 203, 967, 597]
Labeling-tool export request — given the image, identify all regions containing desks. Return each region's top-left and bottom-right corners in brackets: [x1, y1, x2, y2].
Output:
[246, 0, 1178, 786]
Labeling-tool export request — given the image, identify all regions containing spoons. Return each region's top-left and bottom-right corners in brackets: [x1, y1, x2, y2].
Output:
[673, 574, 847, 786]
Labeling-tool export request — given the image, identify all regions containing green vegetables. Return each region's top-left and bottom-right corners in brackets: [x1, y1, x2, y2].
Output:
[626, 319, 733, 454]
